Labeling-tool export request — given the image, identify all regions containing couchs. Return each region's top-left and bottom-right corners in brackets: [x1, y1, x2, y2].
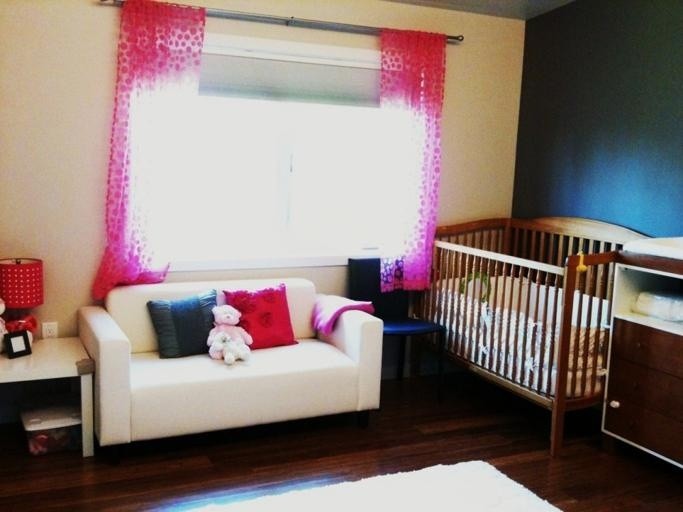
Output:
[73, 275, 387, 459]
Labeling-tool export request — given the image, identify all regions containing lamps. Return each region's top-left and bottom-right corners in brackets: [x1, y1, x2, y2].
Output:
[0, 254, 42, 337]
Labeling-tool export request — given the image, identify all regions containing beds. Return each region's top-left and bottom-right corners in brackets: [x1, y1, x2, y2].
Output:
[411, 213, 651, 458]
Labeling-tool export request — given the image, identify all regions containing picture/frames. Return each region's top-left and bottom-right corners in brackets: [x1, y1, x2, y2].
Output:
[3, 332, 34, 358]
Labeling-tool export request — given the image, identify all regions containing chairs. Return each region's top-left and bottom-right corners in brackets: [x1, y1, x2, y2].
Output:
[343, 251, 450, 406]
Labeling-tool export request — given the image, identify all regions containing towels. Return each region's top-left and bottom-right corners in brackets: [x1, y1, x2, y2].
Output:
[615, 236, 683, 262]
[310, 293, 375, 336]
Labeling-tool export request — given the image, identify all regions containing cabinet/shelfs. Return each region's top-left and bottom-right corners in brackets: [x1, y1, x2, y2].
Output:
[600, 244, 682, 474]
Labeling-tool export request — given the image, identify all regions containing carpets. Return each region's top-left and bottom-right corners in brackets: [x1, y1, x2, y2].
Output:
[173, 456, 568, 512]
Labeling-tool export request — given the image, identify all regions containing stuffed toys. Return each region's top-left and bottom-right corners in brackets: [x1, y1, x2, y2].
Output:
[207, 304, 252, 359]
[459, 271, 491, 305]
[212, 331, 252, 364]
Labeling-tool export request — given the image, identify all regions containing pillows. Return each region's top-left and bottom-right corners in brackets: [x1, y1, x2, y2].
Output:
[221, 282, 297, 350]
[144, 288, 220, 360]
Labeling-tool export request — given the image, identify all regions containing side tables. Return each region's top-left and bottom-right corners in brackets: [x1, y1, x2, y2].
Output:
[1, 330, 97, 466]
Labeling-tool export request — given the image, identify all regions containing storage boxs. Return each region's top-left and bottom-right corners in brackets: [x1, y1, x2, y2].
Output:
[15, 407, 80, 455]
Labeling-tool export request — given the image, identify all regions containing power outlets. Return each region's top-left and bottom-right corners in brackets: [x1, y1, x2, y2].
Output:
[41, 321, 58, 340]
